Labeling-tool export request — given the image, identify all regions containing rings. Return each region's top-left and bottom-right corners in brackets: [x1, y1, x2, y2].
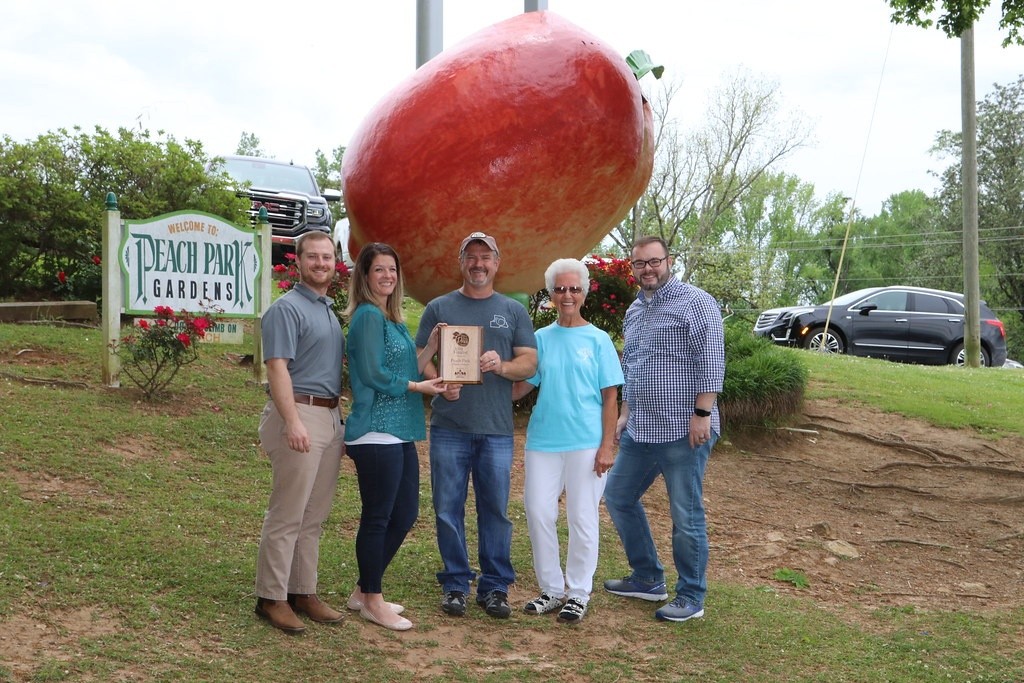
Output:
[492, 360, 495, 365]
[700, 437, 705, 439]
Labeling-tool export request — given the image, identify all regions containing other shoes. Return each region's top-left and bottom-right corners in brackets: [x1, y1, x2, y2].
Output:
[359, 603, 412, 630]
[476, 589, 511, 617]
[441, 591, 467, 615]
[655, 594, 705, 622]
[347, 594, 404, 615]
[604, 576, 668, 602]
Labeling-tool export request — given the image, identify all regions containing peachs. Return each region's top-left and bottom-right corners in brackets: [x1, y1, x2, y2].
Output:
[339, 10, 654, 304]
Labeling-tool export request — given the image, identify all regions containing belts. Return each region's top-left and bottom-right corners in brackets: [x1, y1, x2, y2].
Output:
[270, 393, 340, 409]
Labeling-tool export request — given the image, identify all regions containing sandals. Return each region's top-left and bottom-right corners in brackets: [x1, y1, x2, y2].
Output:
[522, 592, 563, 614]
[556, 597, 587, 624]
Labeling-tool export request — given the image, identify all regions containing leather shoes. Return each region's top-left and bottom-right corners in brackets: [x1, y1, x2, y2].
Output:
[287, 593, 345, 623]
[255, 597, 308, 630]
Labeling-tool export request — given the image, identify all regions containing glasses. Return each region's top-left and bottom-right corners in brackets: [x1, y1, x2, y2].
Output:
[631, 255, 668, 269]
[552, 286, 584, 294]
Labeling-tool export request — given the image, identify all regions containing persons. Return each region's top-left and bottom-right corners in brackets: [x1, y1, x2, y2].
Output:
[337, 242, 447, 631]
[254, 230, 346, 633]
[512, 258, 626, 625]
[414, 231, 538, 618]
[605, 236, 726, 623]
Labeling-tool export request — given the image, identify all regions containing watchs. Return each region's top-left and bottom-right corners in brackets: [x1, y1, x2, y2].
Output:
[694, 408, 711, 417]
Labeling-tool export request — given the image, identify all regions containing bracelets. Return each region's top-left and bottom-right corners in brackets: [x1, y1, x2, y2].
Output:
[340, 419, 345, 425]
[413, 381, 418, 391]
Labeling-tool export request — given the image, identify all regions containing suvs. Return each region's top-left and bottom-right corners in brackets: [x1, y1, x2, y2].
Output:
[199, 155, 342, 265]
[753, 284, 1007, 367]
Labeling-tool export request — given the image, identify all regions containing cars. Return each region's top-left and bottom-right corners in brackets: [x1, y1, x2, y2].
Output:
[331, 215, 355, 274]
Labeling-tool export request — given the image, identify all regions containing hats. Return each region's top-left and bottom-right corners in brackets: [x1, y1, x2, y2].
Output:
[459, 231, 500, 256]
